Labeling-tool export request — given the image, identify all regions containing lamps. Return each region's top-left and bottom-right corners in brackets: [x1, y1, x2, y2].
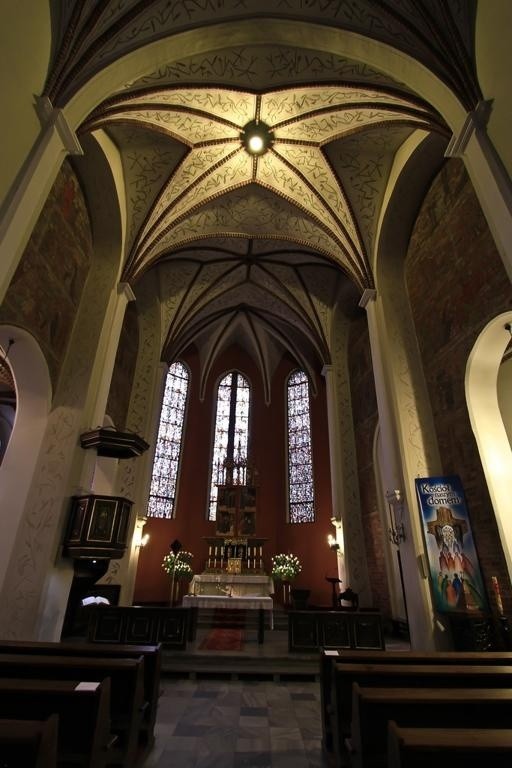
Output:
[385, 491, 407, 545]
[250, 137, 262, 151]
[327, 535, 343, 555]
[134, 534, 150, 549]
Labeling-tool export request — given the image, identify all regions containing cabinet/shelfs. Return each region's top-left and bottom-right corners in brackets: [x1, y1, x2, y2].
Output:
[88, 606, 197, 649]
[287, 608, 386, 653]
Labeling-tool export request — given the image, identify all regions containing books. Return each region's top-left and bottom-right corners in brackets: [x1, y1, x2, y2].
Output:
[82, 595, 110, 607]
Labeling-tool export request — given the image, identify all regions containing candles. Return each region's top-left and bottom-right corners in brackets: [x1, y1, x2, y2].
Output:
[207, 545, 264, 568]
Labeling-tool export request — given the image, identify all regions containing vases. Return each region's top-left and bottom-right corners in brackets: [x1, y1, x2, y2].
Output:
[283, 584, 292, 608]
[170, 576, 181, 605]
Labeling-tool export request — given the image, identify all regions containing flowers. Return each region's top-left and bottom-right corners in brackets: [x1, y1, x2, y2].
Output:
[271, 553, 303, 584]
[161, 552, 192, 576]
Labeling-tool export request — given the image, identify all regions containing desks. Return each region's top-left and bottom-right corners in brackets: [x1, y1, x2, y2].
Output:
[182, 595, 273, 643]
[321, 643, 511, 766]
[191, 571, 275, 598]
[2, 640, 164, 766]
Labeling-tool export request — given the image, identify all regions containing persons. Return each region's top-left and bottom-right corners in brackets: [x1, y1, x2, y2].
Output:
[436, 535, 486, 612]
